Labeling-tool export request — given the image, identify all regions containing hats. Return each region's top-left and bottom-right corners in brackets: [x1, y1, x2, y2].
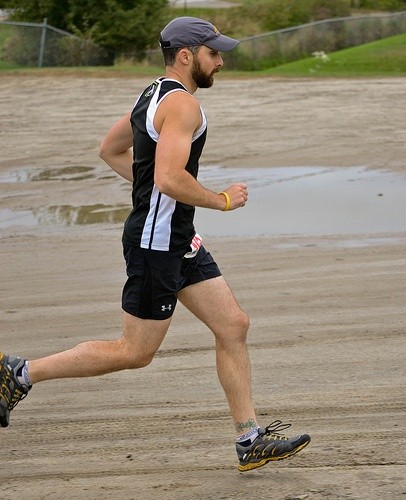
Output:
[158, 17, 240, 52]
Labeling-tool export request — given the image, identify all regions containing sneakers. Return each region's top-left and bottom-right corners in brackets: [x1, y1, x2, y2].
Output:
[235, 419, 311, 470]
[0, 352, 32, 426]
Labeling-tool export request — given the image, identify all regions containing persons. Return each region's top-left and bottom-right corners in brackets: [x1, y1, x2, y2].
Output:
[0, 17, 310, 471]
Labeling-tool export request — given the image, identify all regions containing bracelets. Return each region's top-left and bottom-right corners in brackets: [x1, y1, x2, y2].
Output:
[217, 192, 231, 211]
